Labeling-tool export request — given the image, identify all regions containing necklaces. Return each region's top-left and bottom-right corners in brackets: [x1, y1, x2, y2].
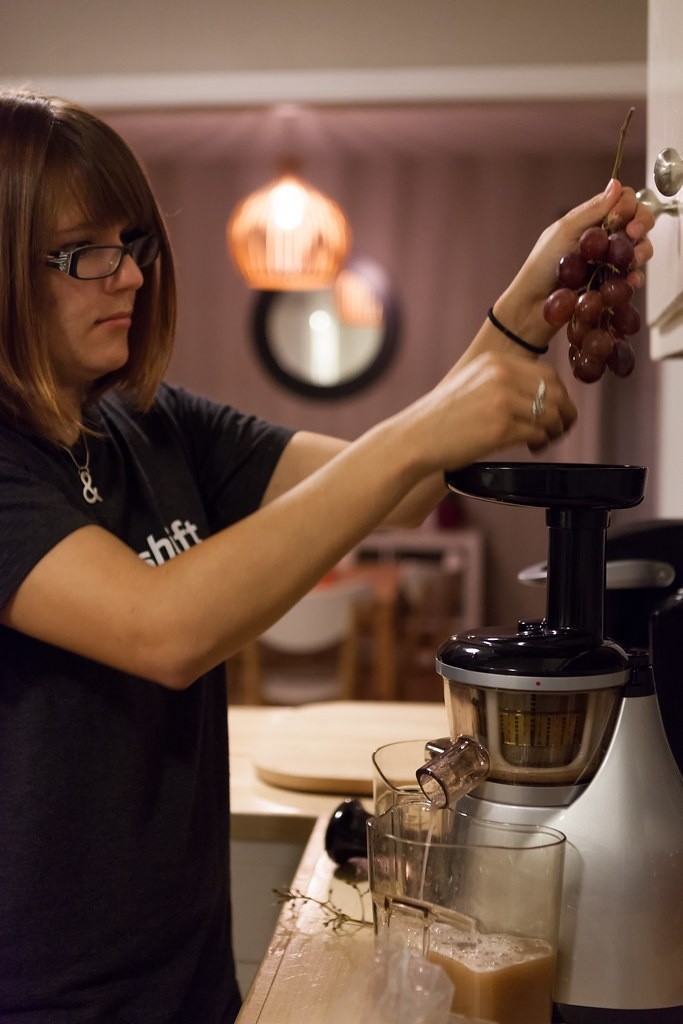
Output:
[53, 430, 103, 504]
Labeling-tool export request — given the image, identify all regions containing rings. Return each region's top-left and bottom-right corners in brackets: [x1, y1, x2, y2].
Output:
[532, 376, 547, 416]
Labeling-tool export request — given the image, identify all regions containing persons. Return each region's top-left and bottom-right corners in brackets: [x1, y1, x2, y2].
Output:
[0, 88, 657, 1024]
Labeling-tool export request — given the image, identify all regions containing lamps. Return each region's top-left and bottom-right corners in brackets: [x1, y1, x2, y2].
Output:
[331, 269, 386, 326]
[226, 103, 351, 292]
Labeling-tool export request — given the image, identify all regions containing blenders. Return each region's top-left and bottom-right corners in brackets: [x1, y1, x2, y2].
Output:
[366, 461, 683, 1024]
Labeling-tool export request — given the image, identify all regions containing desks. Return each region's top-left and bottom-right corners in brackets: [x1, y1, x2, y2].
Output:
[356, 534, 487, 696]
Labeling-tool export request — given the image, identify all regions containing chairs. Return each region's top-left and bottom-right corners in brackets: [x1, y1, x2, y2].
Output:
[239, 577, 370, 708]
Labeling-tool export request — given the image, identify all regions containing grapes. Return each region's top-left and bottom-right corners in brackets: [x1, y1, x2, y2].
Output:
[542, 226, 641, 383]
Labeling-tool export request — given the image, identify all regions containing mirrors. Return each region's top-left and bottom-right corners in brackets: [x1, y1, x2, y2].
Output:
[253, 255, 399, 396]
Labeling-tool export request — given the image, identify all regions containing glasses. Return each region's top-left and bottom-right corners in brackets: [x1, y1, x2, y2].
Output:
[43, 230, 162, 282]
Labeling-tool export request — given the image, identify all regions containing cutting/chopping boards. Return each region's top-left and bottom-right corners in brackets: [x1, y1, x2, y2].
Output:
[246, 700, 451, 794]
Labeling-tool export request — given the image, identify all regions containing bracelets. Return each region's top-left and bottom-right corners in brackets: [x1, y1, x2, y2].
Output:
[487, 307, 549, 355]
